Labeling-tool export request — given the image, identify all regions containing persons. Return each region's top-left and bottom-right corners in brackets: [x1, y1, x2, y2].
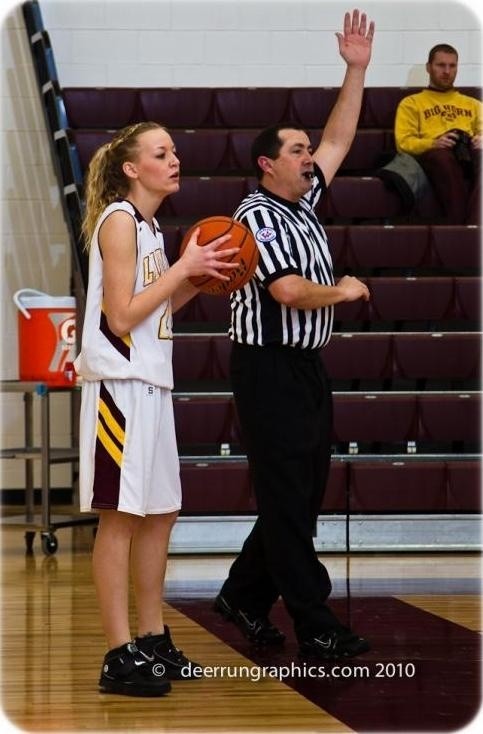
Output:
[379, 44, 481, 225]
[76, 122, 242, 694]
[215, 8, 375, 658]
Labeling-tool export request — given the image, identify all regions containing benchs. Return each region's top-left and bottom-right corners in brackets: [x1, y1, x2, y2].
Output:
[316, 157, 482, 516]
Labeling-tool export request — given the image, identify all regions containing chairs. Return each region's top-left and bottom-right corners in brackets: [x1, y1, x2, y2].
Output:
[347, 85, 403, 157]
[170, 290, 261, 515]
[155, 151, 261, 268]
[61, 85, 341, 150]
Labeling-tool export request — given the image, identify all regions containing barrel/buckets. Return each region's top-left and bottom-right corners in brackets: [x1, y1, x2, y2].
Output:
[13, 287, 78, 389]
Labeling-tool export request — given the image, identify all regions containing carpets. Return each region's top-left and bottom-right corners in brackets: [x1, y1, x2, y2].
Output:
[159, 593, 482, 733]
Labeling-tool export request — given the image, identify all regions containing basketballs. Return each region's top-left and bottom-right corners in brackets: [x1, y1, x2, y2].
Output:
[181, 216, 258, 294]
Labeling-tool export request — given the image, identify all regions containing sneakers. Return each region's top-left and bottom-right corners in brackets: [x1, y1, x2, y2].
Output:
[296, 624, 369, 660]
[133, 623, 204, 680]
[97, 638, 172, 696]
[210, 593, 286, 653]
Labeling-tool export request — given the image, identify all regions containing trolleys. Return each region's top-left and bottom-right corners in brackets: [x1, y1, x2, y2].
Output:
[0, 377, 99, 555]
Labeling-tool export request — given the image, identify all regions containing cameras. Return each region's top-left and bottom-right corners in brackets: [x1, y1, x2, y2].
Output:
[445, 134, 474, 166]
